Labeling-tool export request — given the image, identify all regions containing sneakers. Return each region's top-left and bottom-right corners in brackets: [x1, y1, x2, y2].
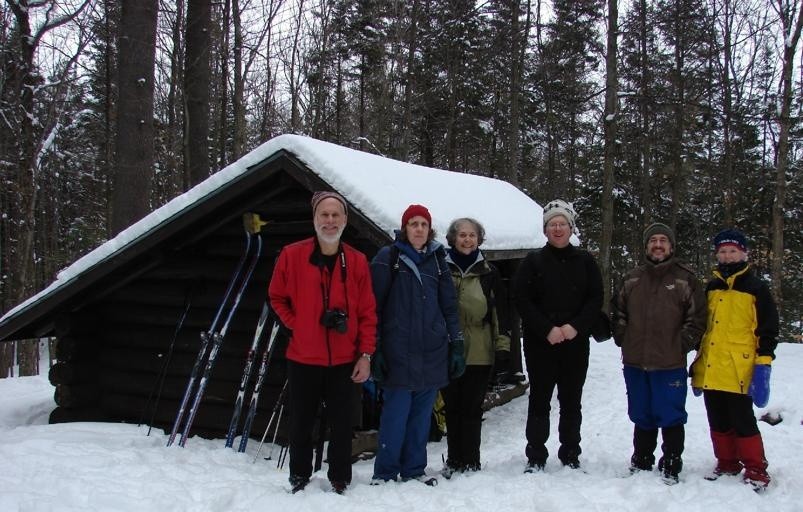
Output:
[329, 481, 347, 495]
[289, 475, 309, 493]
[524, 461, 544, 473]
[660, 470, 678, 485]
[559, 458, 580, 469]
[369, 458, 480, 487]
[706, 466, 770, 492]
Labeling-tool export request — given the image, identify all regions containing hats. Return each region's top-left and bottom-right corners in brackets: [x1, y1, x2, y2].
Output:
[311, 191, 348, 217]
[714, 229, 746, 253]
[642, 223, 675, 251]
[401, 204, 431, 230]
[543, 200, 575, 232]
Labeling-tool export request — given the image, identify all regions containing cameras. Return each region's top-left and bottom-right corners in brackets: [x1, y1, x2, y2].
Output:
[320, 307, 347, 334]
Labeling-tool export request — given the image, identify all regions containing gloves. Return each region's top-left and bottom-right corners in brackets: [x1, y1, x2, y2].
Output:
[495, 350, 511, 375]
[747, 356, 772, 407]
[449, 341, 465, 379]
[370, 350, 391, 382]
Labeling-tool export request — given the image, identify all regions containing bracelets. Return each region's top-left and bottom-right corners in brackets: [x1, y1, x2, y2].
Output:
[362, 352, 371, 361]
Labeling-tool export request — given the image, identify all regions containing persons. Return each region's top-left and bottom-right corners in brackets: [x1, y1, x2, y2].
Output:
[267, 191, 378, 495]
[514, 197, 611, 474]
[371, 205, 465, 486]
[437, 218, 512, 477]
[688, 229, 779, 493]
[610, 224, 709, 485]
